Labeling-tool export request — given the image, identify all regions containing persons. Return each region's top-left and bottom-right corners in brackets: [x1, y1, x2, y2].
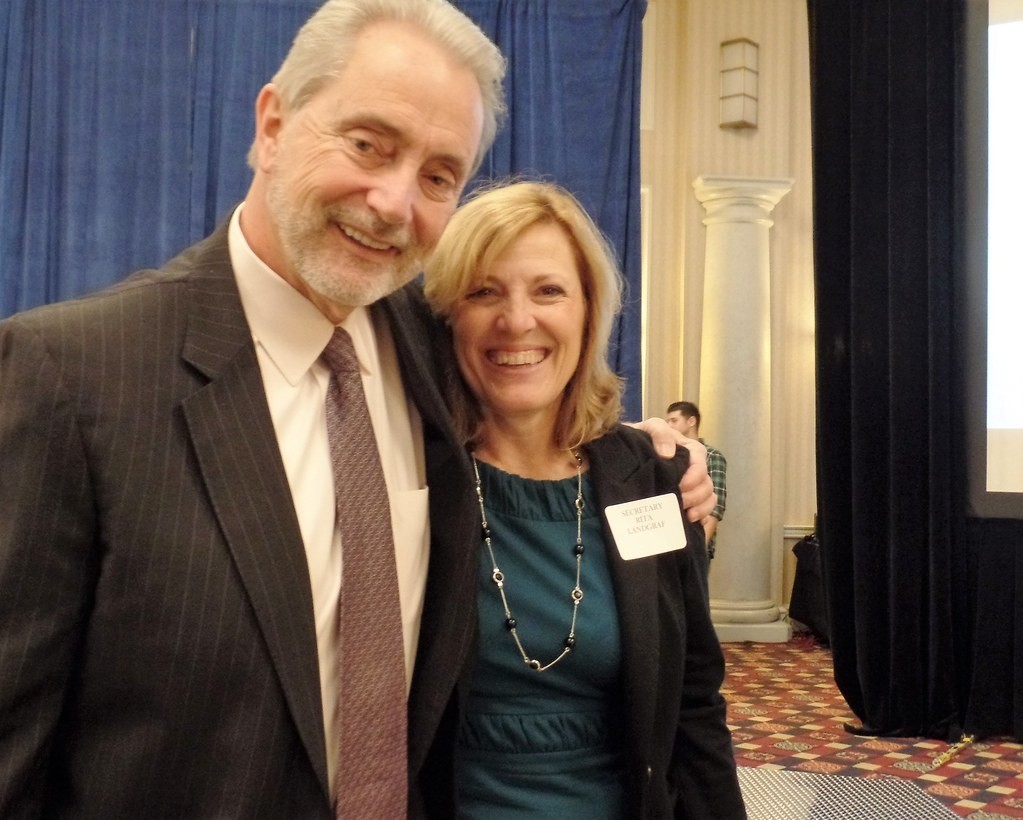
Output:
[665, 402, 728, 575]
[406, 181, 753, 820]
[0, 1, 718, 820]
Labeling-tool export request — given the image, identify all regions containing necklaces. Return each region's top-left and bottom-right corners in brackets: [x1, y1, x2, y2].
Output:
[464, 439, 585, 672]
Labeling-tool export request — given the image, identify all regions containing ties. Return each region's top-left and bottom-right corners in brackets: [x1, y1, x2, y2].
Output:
[321, 326, 408, 820]
